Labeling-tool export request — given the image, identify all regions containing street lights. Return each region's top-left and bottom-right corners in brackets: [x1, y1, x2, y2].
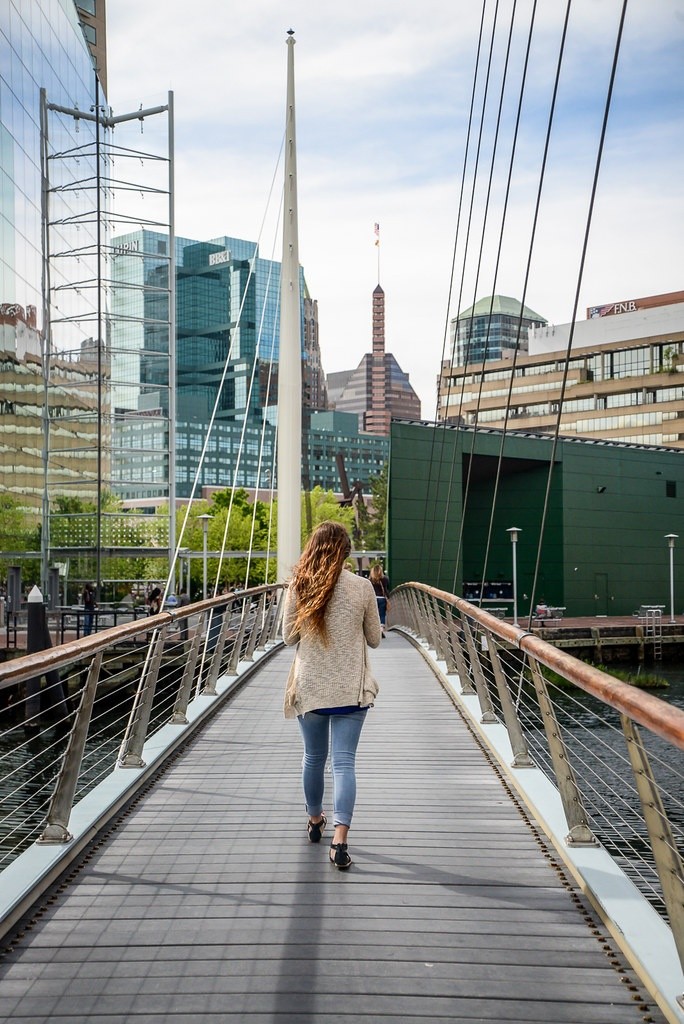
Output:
[196, 514, 215, 599]
[663, 533, 679, 624]
[505, 527, 522, 628]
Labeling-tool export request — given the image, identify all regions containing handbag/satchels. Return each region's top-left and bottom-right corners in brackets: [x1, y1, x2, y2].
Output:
[386, 598, 391, 610]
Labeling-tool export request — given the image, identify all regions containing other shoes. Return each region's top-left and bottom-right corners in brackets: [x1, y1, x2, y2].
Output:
[381, 630, 386, 639]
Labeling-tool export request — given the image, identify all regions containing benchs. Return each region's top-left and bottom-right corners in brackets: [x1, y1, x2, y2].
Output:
[534, 607, 567, 627]
[632, 605, 666, 625]
[474, 607, 508, 628]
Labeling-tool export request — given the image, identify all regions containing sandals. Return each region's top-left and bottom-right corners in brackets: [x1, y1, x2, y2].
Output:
[329, 843, 352, 872]
[307, 813, 327, 843]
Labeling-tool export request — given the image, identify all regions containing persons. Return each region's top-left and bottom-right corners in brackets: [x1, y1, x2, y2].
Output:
[536, 599, 553, 627]
[82, 585, 98, 636]
[145, 587, 192, 640]
[368, 565, 390, 639]
[0, 591, 7, 623]
[206, 583, 271, 614]
[282, 520, 381, 867]
